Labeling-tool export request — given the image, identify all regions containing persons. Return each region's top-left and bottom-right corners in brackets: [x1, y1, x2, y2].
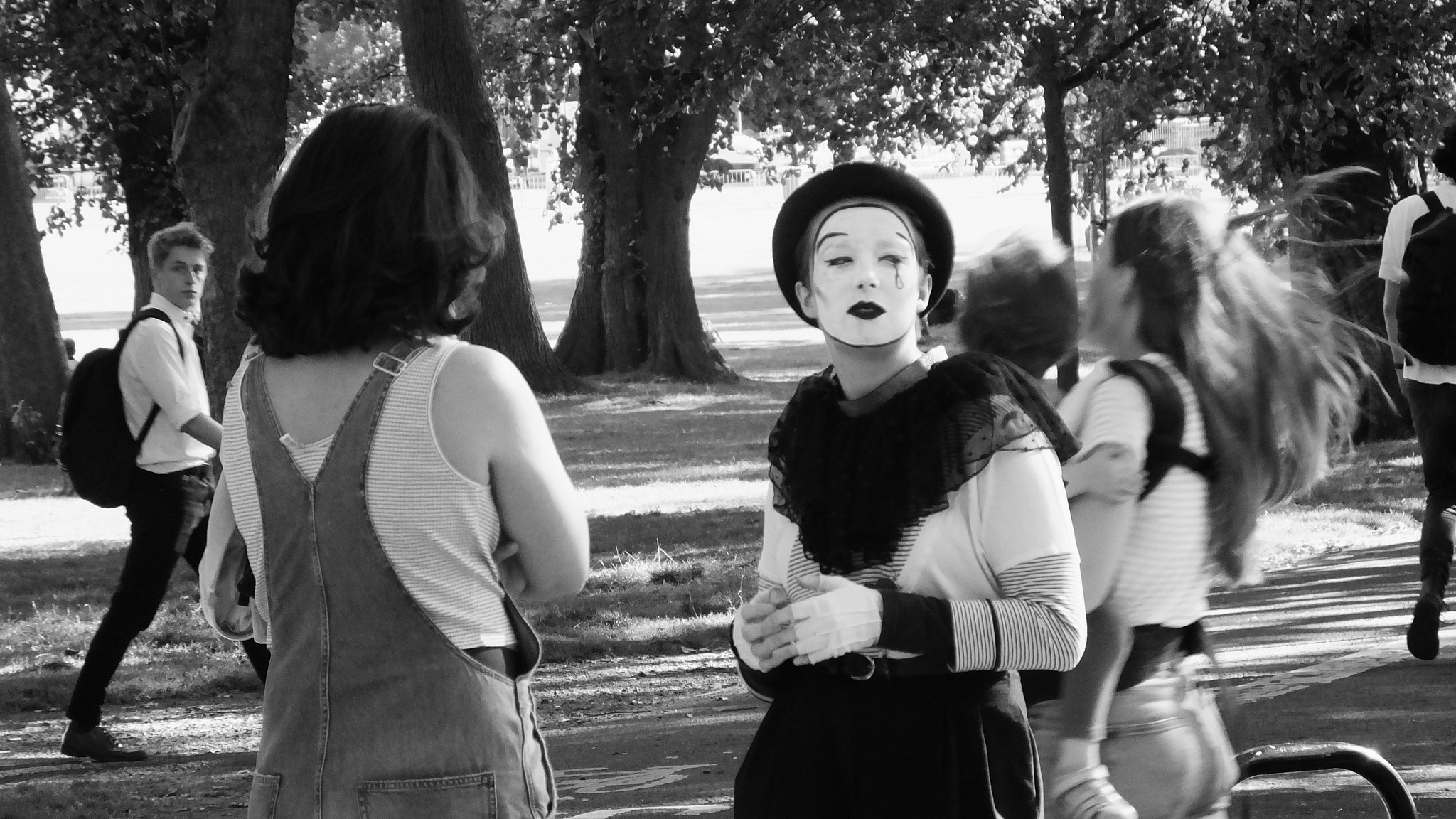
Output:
[1376, 118, 1456, 660]
[57, 218, 272, 763]
[952, 233, 1153, 507]
[194, 101, 590, 819]
[724, 161, 1089, 819]
[1019, 166, 1411, 819]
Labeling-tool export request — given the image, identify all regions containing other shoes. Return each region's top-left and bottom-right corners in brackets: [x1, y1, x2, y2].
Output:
[1407, 596, 1440, 659]
[1049, 762, 1138, 819]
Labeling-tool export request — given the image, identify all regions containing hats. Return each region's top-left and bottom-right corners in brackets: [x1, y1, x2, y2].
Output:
[772, 160, 957, 332]
[1433, 125, 1456, 180]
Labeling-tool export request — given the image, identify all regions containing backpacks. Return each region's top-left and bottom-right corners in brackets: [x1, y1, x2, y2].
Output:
[62, 308, 183, 509]
[1395, 189, 1455, 366]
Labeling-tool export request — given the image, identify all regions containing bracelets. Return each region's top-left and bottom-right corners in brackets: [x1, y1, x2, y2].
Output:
[1393, 363, 1404, 370]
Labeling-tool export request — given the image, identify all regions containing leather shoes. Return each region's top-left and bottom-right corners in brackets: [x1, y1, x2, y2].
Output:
[59, 722, 149, 763]
